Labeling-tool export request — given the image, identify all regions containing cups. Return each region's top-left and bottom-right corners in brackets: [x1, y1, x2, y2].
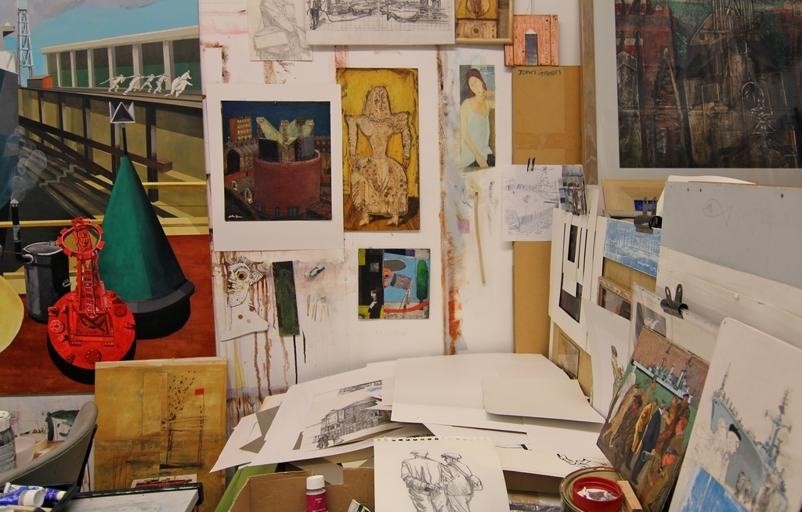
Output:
[22, 242, 70, 323]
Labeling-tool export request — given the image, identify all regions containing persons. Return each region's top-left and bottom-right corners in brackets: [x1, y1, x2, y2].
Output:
[461, 68, 496, 169]
[401, 447, 473, 512]
[601, 371, 690, 508]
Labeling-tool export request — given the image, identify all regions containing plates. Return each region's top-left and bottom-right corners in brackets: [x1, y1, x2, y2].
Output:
[560, 466, 619, 512]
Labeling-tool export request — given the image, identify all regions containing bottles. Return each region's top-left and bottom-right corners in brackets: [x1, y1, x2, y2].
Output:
[0, 410, 15, 475]
